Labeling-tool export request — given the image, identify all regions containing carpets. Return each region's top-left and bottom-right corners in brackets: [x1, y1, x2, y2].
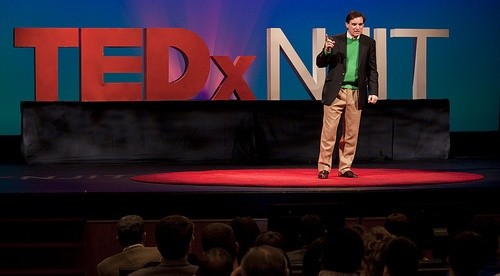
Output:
[130, 169, 484, 187]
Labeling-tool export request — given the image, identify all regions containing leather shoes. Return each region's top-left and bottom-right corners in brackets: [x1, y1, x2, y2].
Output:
[318, 170, 328, 179]
[340, 171, 357, 178]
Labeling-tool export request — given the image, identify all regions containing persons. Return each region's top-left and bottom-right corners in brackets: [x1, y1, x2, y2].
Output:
[316, 10, 378, 178]
[97, 203, 500, 276]
